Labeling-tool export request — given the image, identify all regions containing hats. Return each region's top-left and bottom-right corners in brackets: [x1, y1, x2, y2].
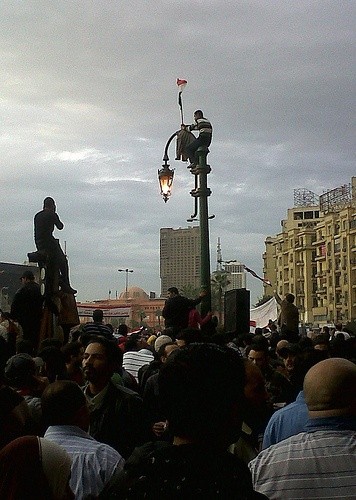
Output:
[5, 353, 44, 380]
[18, 271, 34, 280]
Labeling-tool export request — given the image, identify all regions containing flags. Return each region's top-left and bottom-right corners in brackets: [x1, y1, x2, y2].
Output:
[177, 78, 188, 110]
[243, 264, 273, 287]
[250, 296, 278, 334]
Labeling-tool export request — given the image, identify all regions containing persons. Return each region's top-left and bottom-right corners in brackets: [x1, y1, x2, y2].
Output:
[273, 291, 299, 338]
[56, 281, 81, 341]
[33, 197, 78, 293]
[162, 286, 206, 336]
[0, 310, 356, 500]
[187, 109, 212, 172]
[10, 270, 45, 358]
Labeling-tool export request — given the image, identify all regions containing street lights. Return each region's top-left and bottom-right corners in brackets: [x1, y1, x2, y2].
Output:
[157, 128, 212, 318]
[117, 268, 133, 298]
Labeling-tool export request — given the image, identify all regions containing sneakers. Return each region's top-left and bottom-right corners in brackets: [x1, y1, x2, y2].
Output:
[187, 163, 196, 168]
[61, 284, 77, 293]
